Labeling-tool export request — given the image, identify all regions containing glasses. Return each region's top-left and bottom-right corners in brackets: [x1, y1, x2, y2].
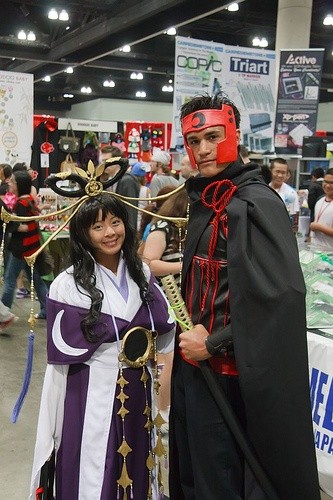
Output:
[322, 180, 333, 185]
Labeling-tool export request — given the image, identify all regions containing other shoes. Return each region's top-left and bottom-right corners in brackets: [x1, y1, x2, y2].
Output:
[16, 288, 31, 298]
[0, 313, 19, 334]
[32, 314, 46, 319]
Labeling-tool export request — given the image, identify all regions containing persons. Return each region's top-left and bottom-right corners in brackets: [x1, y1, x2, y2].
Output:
[269, 157, 299, 235]
[98, 145, 191, 301]
[28, 192, 177, 500]
[307, 169, 327, 222]
[170, 91, 321, 500]
[0, 163, 56, 328]
[308, 168, 333, 243]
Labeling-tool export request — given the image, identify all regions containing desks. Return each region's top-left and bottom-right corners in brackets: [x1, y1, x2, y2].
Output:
[41, 231, 70, 281]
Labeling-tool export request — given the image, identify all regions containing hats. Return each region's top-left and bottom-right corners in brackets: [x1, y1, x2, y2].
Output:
[151, 146, 171, 165]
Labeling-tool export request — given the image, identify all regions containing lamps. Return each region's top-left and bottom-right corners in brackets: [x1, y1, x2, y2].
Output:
[16, 3, 333, 99]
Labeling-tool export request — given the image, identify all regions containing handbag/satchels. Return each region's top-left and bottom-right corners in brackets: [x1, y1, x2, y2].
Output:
[127, 128, 166, 166]
[58, 122, 80, 153]
[111, 133, 126, 152]
[60, 154, 78, 171]
[38, 239, 54, 282]
